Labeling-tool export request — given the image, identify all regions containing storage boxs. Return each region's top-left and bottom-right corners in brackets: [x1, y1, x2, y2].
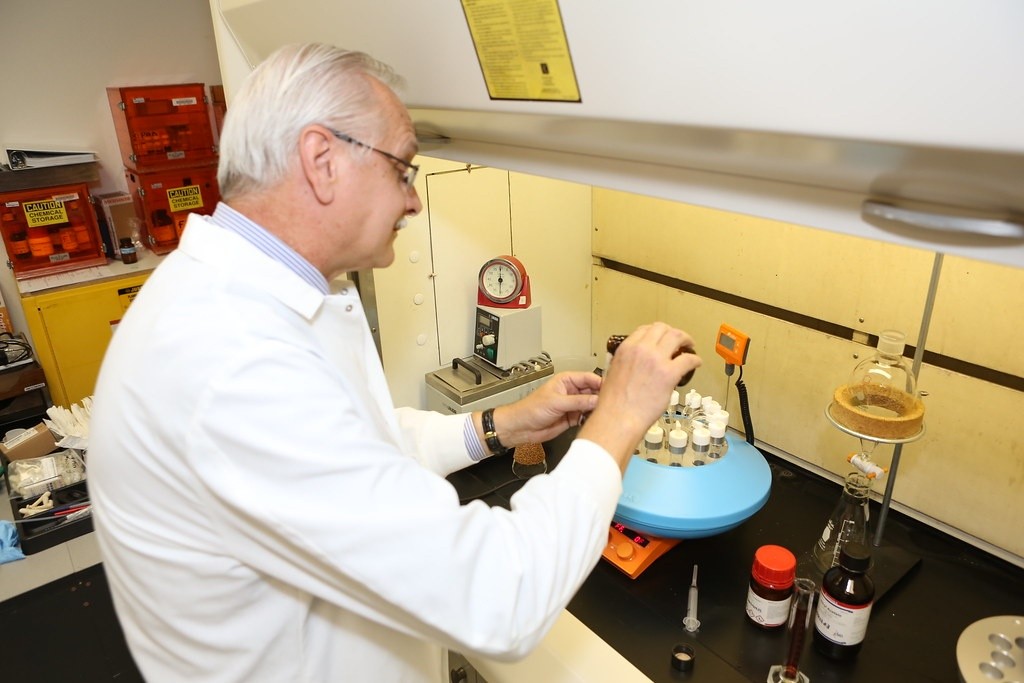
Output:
[0, 421, 59, 464]
[15, 457, 64, 501]
[0, 82, 226, 281]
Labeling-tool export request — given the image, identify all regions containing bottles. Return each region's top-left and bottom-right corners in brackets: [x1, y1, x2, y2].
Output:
[745, 545, 797, 631]
[815, 472, 874, 575]
[846, 328, 918, 417]
[814, 541, 875, 663]
[0, 202, 91, 264]
[119, 237, 138, 264]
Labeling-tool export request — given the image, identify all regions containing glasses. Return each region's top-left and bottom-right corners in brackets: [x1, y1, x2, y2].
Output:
[326, 127, 419, 192]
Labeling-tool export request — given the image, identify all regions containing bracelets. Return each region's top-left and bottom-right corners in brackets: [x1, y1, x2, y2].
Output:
[482, 408, 508, 455]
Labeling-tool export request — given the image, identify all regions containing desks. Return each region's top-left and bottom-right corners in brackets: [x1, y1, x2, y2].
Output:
[13, 249, 170, 410]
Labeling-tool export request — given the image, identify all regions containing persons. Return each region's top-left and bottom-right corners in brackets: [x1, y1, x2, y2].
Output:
[86, 40, 703, 683]
